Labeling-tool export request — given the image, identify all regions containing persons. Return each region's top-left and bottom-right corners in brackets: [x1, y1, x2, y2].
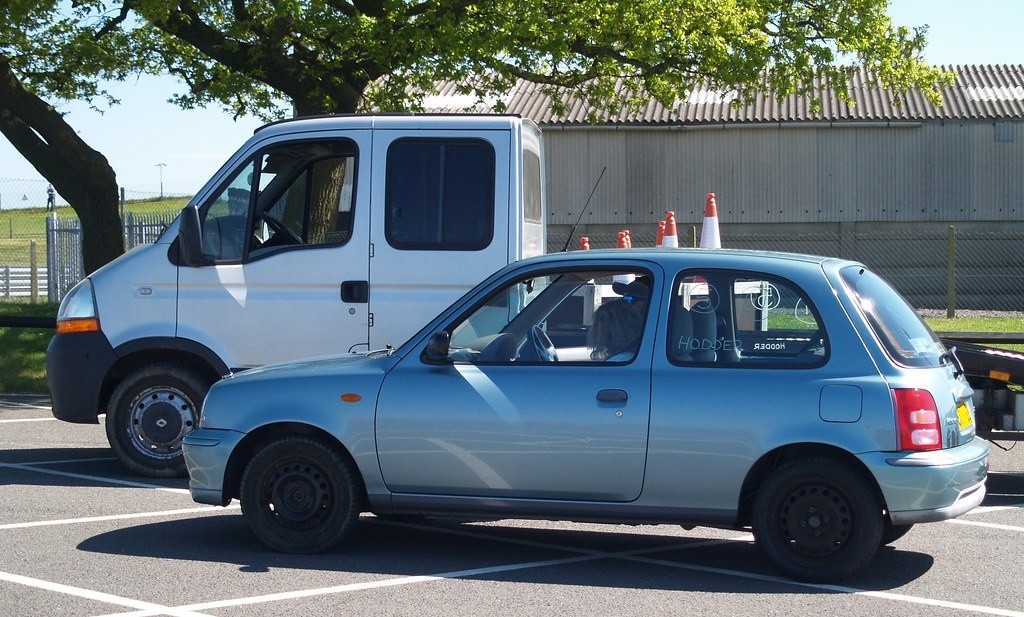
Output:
[46, 184, 56, 212]
[612, 275, 652, 311]
[591, 298, 641, 361]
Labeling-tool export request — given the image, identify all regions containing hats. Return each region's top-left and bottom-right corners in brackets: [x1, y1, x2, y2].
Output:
[612, 281, 650, 297]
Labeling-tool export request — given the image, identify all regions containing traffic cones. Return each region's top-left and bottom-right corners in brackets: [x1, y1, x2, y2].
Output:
[662, 211, 679, 248]
[656, 221, 667, 247]
[611, 229, 636, 285]
[579, 236, 594, 284]
[693, 193, 721, 284]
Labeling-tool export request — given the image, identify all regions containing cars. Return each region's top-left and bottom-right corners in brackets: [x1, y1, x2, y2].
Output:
[184, 247, 991, 584]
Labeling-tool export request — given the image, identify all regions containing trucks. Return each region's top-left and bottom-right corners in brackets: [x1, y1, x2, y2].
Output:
[45, 111, 1024, 480]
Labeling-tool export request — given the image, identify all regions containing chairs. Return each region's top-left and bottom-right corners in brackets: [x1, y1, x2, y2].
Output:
[667, 299, 718, 362]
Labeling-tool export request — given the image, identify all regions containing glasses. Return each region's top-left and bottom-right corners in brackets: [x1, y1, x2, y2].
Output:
[623, 295, 643, 304]
